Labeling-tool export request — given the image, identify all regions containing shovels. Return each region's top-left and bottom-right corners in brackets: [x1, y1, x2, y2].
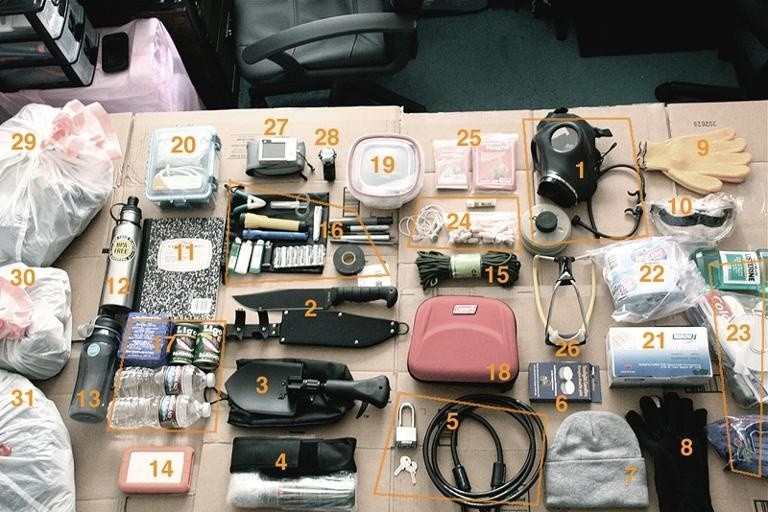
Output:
[224, 361, 389, 416]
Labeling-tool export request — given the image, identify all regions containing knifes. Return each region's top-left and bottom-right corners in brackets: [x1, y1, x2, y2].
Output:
[232, 287, 399, 310]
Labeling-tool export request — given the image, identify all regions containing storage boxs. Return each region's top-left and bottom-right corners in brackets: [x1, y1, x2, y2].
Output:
[0, 0, 101, 92]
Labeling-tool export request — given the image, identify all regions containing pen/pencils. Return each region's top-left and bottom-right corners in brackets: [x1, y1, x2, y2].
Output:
[329, 217, 393, 241]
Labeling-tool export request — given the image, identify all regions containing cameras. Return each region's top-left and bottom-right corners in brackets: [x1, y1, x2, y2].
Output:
[257, 137, 299, 165]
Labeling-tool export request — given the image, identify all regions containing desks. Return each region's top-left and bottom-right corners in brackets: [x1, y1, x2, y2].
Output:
[2, 95, 767, 512]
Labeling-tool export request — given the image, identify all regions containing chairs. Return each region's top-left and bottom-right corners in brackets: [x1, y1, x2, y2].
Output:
[231, 1, 426, 111]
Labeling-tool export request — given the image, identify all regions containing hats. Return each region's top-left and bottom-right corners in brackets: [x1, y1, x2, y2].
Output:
[544, 410, 649, 512]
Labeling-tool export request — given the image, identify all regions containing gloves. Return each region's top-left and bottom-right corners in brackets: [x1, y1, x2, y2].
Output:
[637, 128, 752, 195]
[625, 392, 714, 512]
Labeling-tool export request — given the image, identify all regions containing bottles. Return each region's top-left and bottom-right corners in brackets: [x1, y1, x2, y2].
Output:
[115, 364, 217, 399]
[67, 311, 124, 424]
[107, 396, 211, 435]
[100, 195, 143, 316]
